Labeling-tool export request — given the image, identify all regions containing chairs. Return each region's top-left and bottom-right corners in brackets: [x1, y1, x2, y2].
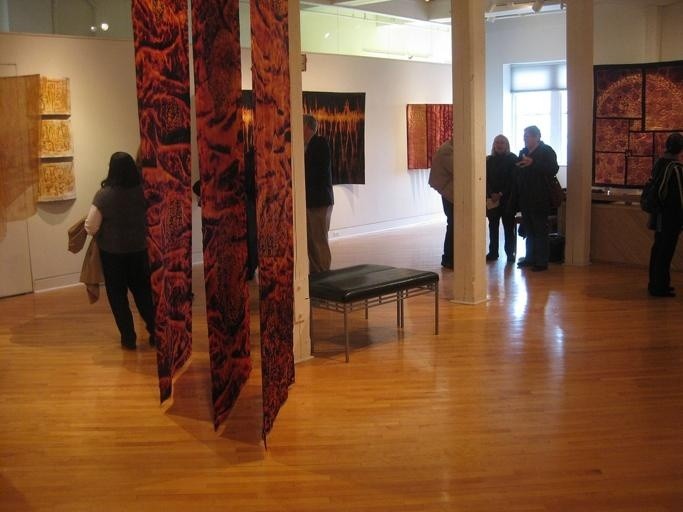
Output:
[648, 283, 676, 297]
[120, 335, 156, 349]
[485, 251, 548, 271]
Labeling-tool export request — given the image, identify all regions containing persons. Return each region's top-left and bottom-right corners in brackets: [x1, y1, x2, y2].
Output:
[428, 135, 453, 270]
[85, 151, 154, 349]
[192, 179, 200, 195]
[303, 114, 334, 275]
[486, 134, 519, 264]
[245, 144, 259, 288]
[639, 133, 683, 297]
[516, 125, 565, 272]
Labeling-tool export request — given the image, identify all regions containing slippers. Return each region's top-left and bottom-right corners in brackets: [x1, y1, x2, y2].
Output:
[640, 178, 661, 213]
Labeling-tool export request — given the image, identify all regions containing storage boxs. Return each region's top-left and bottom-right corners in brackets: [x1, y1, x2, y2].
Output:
[309, 265, 440, 362]
